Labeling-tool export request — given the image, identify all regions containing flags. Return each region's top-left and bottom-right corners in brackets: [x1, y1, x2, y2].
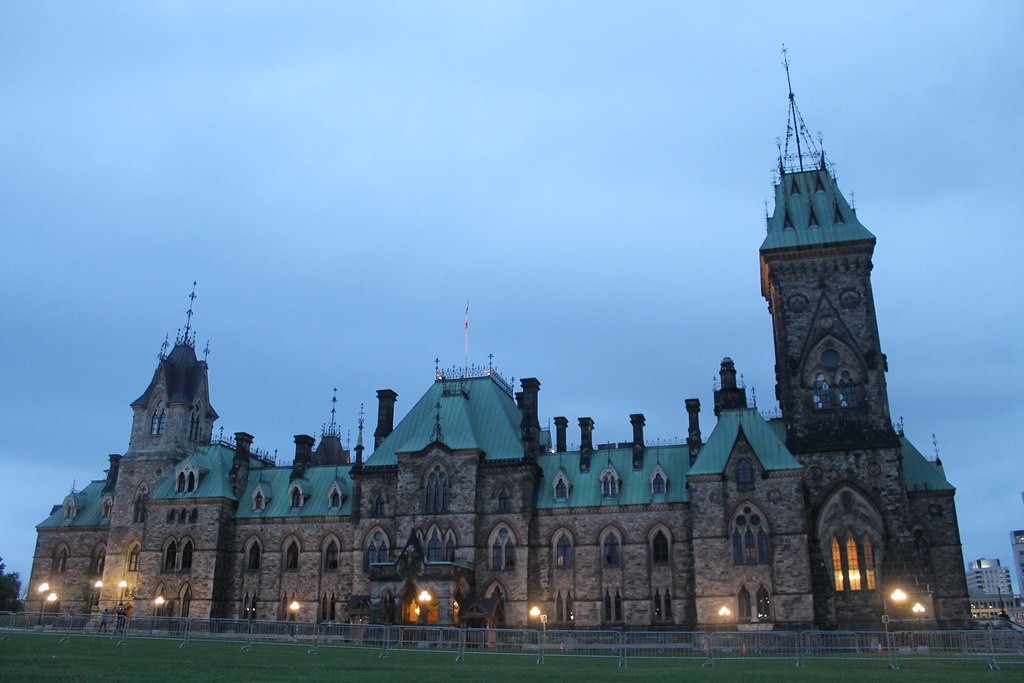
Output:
[465, 301, 469, 329]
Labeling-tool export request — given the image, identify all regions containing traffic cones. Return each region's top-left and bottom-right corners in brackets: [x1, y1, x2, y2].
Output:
[703, 643, 708, 653]
[878, 644, 883, 655]
[741, 644, 747, 653]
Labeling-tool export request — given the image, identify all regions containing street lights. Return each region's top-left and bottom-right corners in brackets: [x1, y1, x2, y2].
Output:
[718, 605, 732, 646]
[530, 607, 541, 644]
[153, 595, 164, 633]
[891, 588, 908, 646]
[289, 602, 299, 638]
[912, 603, 926, 647]
[39, 582, 58, 627]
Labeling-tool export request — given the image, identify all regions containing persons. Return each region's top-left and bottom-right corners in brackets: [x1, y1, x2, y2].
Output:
[116, 603, 127, 635]
[97, 608, 110, 634]
